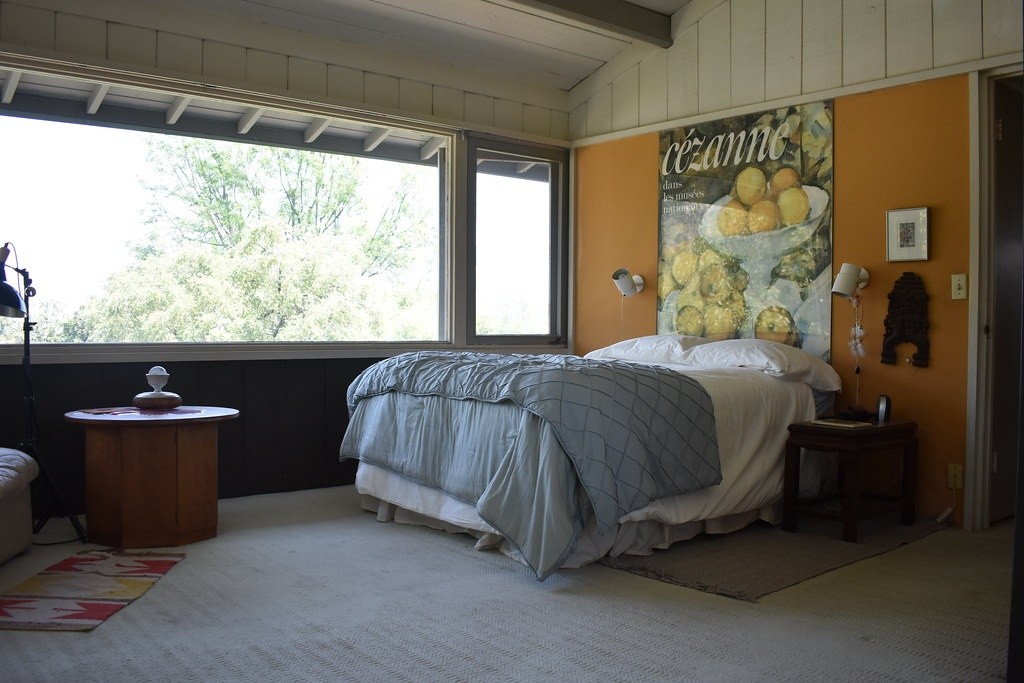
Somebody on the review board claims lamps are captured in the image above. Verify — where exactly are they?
[831,263,870,297]
[0,241,88,545]
[611,268,645,299]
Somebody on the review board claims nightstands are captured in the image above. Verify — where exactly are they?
[782,417,917,542]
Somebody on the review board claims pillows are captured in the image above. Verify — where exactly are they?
[678,338,842,392]
[582,332,695,364]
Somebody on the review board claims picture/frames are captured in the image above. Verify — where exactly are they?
[886,207,930,262]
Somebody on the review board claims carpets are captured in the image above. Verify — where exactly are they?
[0,549,186,634]
[589,512,952,606]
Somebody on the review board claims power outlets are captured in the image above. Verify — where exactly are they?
[950,274,968,300]
[948,461,966,491]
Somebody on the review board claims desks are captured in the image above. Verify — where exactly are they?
[65,406,240,550]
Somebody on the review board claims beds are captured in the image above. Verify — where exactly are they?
[338,331,842,581]
